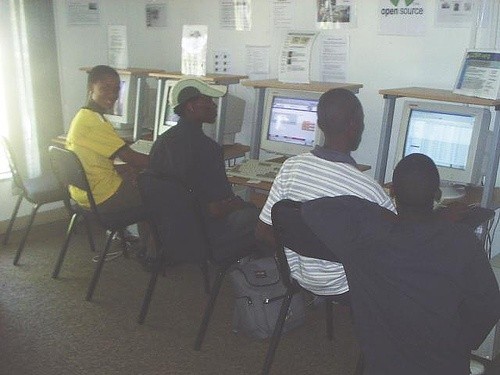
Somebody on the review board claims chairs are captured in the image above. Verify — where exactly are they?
[1,135,63,263]
[133,168,226,348]
[48,146,158,299]
[260,200,352,375]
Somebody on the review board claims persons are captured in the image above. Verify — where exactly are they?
[64,65,158,265]
[253,88,500,375]
[148,78,277,261]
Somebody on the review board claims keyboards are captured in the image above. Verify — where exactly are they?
[130,139,155,156]
[229,159,283,184]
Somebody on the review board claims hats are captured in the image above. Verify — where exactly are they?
[168,76,228,114]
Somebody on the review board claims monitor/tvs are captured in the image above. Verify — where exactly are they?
[260,86,327,157]
[100,73,148,130]
[384,96,492,200]
[158,79,246,147]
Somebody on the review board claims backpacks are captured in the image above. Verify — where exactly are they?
[229,252,306,342]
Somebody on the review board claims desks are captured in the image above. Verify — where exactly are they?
[48,129,499,227]
